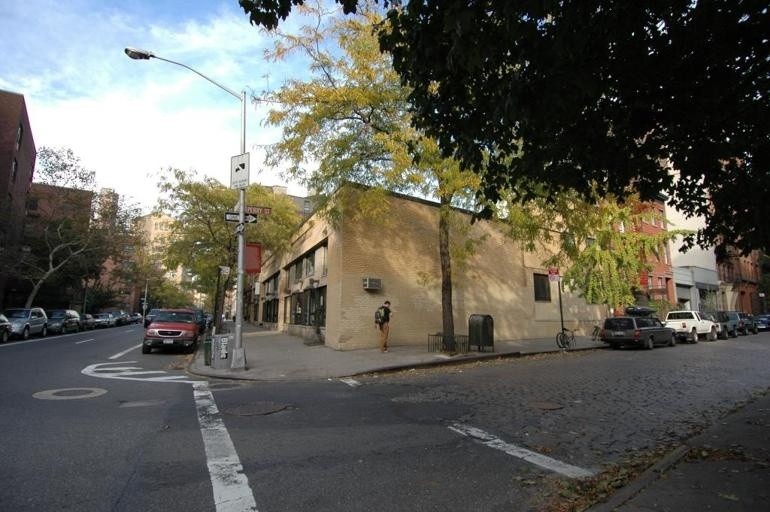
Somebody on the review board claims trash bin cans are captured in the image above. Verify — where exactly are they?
[468,313,495,352]
[211,334,233,369]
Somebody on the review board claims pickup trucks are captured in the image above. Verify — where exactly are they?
[665,310,722,345]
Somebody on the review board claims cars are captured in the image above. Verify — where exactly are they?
[0,312,13,344]
[707,310,770,340]
[81,310,143,331]
[142,308,214,355]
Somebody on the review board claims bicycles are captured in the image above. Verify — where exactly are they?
[592,325,602,341]
[556,328,580,349]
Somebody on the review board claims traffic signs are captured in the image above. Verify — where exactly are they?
[123,45,251,370]
[225,197,271,235]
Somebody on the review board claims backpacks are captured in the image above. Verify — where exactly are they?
[374,306,386,324]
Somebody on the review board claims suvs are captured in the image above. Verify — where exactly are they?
[600,316,677,351]
[45,309,81,335]
[5,306,48,340]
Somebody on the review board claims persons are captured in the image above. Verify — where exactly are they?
[374,300,393,352]
